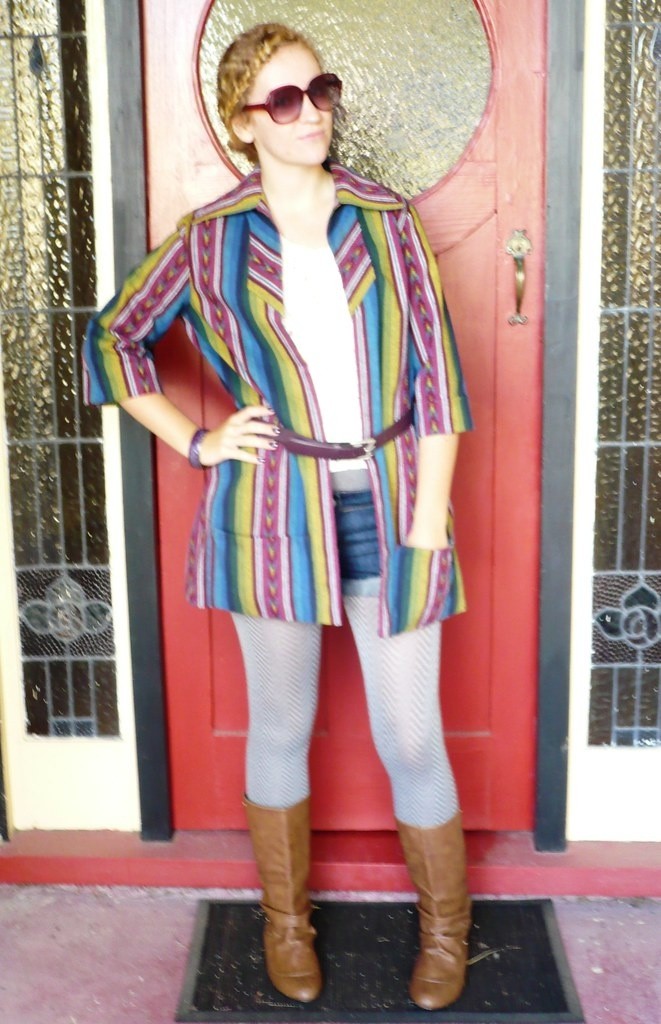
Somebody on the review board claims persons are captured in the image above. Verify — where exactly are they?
[81,24,472,1010]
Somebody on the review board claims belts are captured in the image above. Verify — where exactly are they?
[276,410,414,461]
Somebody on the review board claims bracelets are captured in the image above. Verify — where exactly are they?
[189,429,207,470]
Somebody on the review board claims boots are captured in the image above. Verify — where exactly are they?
[243,793,322,1003]
[394,808,473,1011]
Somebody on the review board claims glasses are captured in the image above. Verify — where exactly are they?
[242,74,343,123]
